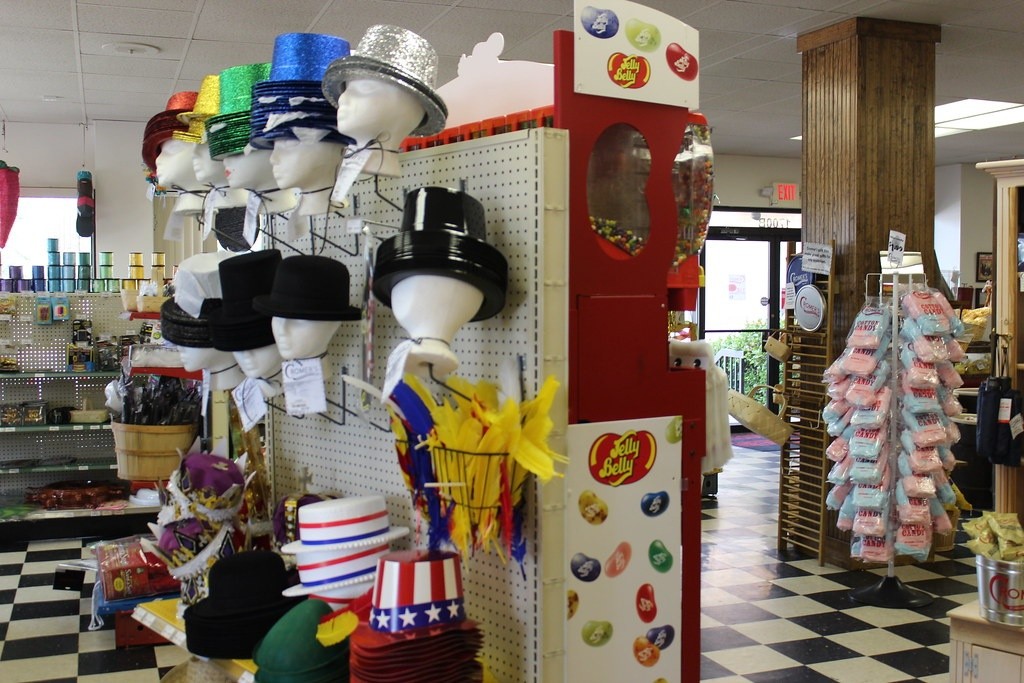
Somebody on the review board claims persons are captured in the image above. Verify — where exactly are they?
[265,99,349,218]
[210,248,289,399]
[322,25,448,183]
[222,114,298,216]
[377,185,507,383]
[162,249,250,393]
[188,121,250,211]
[270,255,360,377]
[155,121,211,215]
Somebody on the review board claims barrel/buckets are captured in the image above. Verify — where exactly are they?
[975,554,1024,629]
[111,420,199,481]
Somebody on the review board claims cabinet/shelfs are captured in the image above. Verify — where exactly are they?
[0,290,232,525]
[950,158,1023,683]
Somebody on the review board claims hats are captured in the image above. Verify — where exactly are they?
[344,547,485,683]
[320,24,448,136]
[253,597,349,683]
[269,490,339,556]
[182,552,307,659]
[171,75,220,144]
[159,252,226,348]
[144,519,234,580]
[250,32,358,149]
[206,249,283,351]
[141,91,199,173]
[204,62,272,162]
[281,493,411,614]
[252,254,362,321]
[372,186,509,322]
[166,435,255,519]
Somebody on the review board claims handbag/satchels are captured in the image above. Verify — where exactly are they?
[764,329,793,363]
[726,384,794,445]
